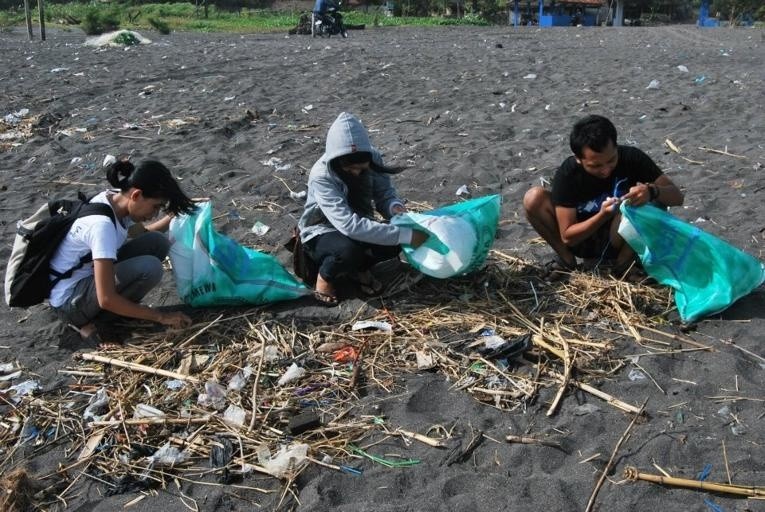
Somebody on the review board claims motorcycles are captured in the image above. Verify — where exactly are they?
[313,1,349,38]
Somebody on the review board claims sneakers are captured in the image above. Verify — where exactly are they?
[113,318,154,327]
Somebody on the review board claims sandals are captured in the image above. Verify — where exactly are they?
[615,261,654,285]
[544,257,577,285]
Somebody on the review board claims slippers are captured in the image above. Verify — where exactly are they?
[313,278,339,307]
[346,272,385,297]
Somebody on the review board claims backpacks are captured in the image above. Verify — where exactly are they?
[4,188,117,308]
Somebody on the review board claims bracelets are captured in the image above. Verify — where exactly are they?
[648,182,659,202]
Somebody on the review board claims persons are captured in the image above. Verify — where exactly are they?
[523,113,684,281]
[282,112,429,309]
[46,160,201,353]
[311,0,339,39]
